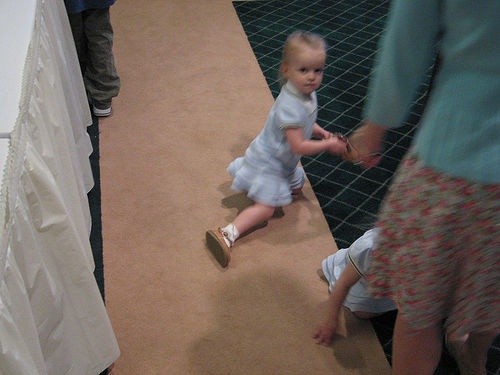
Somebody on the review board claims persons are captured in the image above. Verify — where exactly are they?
[65,0,121,117]
[348,0,500,375]
[309,227,398,347]
[205,31,351,267]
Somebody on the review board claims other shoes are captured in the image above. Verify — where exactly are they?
[92,104,112,117]
[206,230,231,269]
